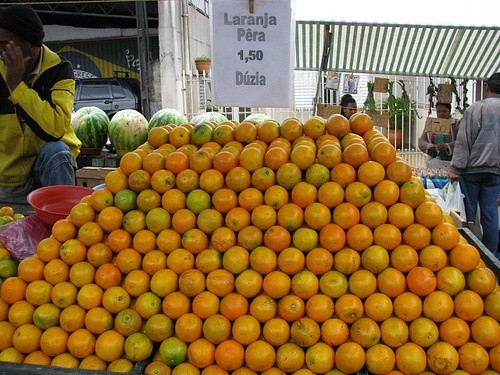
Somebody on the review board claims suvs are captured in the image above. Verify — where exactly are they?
[73,77,141,118]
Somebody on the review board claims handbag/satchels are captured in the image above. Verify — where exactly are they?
[436,181,466,221]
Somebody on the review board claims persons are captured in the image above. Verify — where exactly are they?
[447,72,500,256]
[418,102,461,162]
[0,3,83,198]
[340,94,357,120]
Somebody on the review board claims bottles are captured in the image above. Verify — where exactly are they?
[411,164,449,188]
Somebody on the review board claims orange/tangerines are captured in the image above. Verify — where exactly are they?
[0,112,500,375]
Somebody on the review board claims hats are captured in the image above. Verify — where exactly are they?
[0,4,45,48]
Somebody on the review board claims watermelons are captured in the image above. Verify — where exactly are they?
[189,112,229,124]
[244,113,271,123]
[71,106,110,148]
[108,109,148,156]
[148,108,188,130]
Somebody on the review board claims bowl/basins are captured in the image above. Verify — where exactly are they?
[27,186,94,224]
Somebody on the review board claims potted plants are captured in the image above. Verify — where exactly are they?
[381,95,410,150]
[195,56,211,74]
